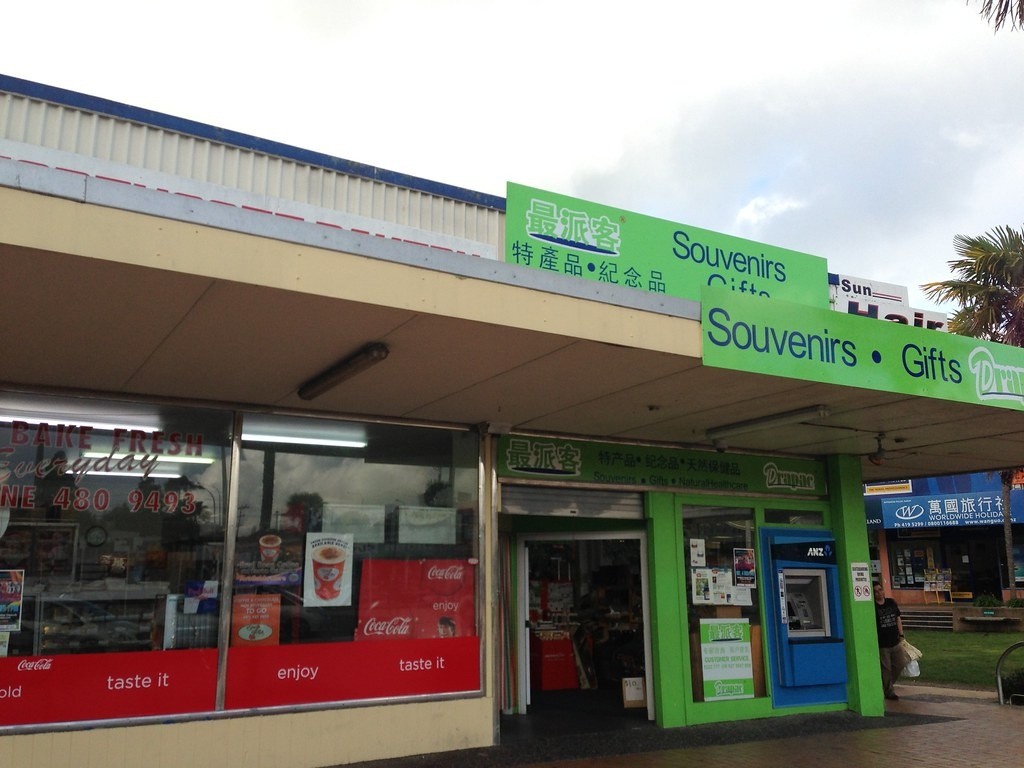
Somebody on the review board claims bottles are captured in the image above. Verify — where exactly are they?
[542,611,570,626]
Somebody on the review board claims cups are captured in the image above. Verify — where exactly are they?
[260,535,281,562]
[309,545,347,601]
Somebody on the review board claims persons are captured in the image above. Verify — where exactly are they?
[438,616,456,638]
[872,580,907,701]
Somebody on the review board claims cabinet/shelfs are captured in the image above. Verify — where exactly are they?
[0,521,170,656]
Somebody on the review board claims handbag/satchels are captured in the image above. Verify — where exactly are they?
[900,639,922,663]
[900,660,920,677]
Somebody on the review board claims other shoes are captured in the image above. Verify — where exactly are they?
[885,694,898,700]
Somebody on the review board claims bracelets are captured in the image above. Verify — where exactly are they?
[899,634,905,638]
